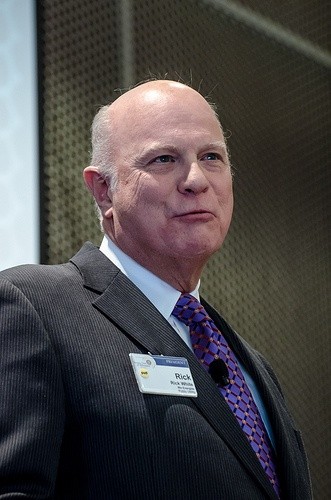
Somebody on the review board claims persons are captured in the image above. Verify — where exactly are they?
[0,80,313,500]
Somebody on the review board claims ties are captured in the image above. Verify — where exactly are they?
[172,293,285,500]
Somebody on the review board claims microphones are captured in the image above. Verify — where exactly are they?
[208,358,230,386]
